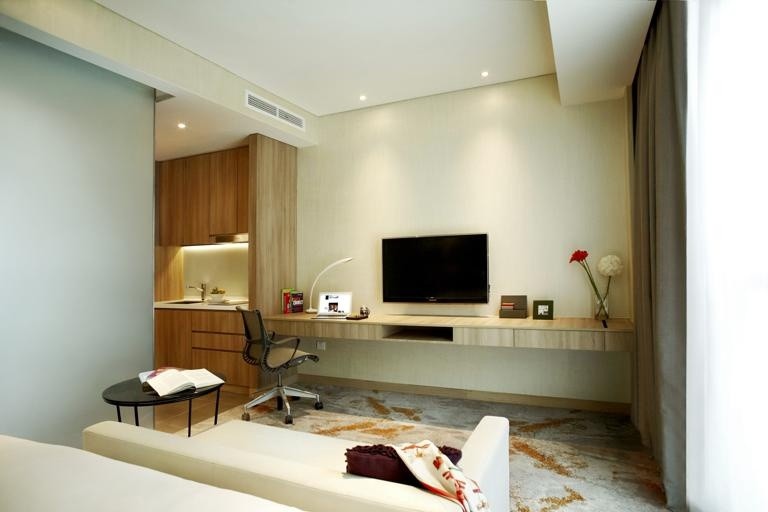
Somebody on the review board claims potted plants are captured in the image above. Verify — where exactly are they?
[208,287,226,303]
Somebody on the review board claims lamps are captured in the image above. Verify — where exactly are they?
[304,258,352,314]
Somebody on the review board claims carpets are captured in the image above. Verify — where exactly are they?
[174,384,686,512]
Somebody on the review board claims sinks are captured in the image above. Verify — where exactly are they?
[167,301,201,304]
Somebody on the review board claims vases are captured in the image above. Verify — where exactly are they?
[593,295,609,321]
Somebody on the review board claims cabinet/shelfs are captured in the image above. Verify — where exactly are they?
[153,308,191,370]
[155,145,209,246]
[263,310,633,355]
[190,298,252,396]
[210,147,249,238]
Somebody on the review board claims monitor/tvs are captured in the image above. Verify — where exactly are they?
[381,232,490,304]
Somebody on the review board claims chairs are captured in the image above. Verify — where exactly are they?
[234,305,325,424]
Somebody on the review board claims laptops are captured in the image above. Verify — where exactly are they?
[311,291,353,320]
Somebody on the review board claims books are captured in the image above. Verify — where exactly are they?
[147,368,225,397]
[146,367,185,380]
[139,371,155,387]
[282,288,304,314]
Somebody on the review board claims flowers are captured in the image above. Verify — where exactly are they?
[569,249,624,318]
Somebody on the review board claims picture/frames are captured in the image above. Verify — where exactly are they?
[533,300,553,320]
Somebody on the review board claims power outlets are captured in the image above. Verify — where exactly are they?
[316,341,326,351]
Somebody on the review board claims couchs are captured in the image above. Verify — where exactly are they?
[82,415,510,512]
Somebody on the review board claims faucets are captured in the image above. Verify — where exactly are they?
[189,283,205,302]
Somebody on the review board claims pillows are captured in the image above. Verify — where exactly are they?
[344,443,462,485]
